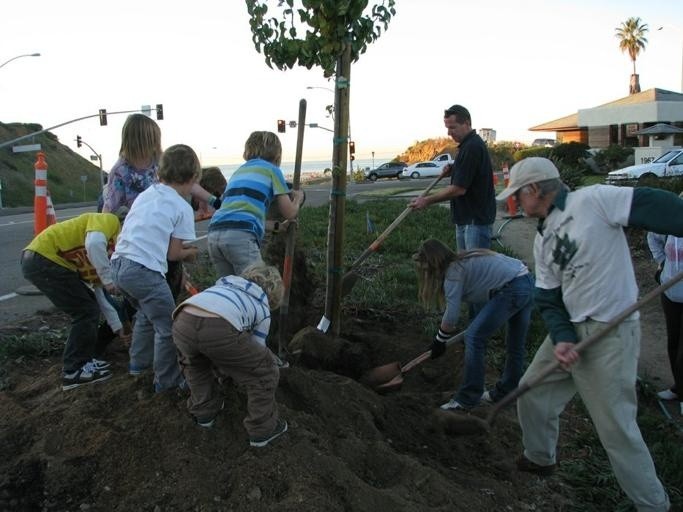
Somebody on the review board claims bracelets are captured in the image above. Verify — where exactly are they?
[271,222,279,235]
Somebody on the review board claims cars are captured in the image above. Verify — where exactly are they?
[362,153,455,181]
[602,149,682,185]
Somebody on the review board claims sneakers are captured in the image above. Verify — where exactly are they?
[62,359,288,447]
[440,392,497,411]
[658,388,683,415]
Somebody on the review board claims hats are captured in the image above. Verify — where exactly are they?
[496,157,560,201]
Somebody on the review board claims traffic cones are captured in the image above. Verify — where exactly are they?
[44,188,55,228]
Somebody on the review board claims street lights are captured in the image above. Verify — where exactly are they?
[0,51,40,74]
[306,85,354,184]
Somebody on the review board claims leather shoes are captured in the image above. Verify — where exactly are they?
[490,454,557,479]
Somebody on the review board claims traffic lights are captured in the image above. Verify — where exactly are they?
[350,156,354,160]
[349,142,353,153]
[277,120,282,133]
[77,133,82,148]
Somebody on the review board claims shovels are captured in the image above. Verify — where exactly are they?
[637,376,682,432]
[274,98,307,386]
[369,331,465,390]
[435,272,683,435]
[340,164,451,297]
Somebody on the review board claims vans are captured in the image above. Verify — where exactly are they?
[188,165,226,212]
[531,139,560,147]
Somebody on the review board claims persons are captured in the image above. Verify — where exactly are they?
[413,237,533,410]
[207,130,305,282]
[404,103,497,321]
[494,156,682,512]
[644,190,683,420]
[19,114,226,392]
[171,262,288,448]
